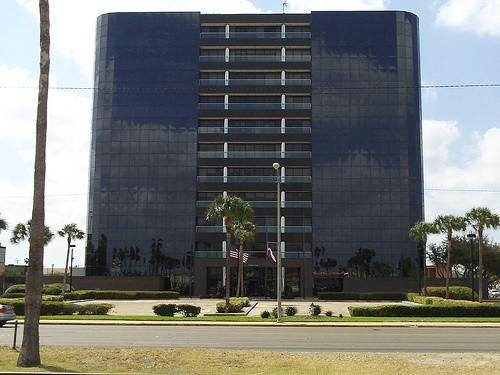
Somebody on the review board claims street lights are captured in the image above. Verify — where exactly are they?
[467,234,476,302]
[70,245,76,292]
[273,163,281,323]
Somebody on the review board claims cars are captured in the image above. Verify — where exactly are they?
[0,304,16,327]
